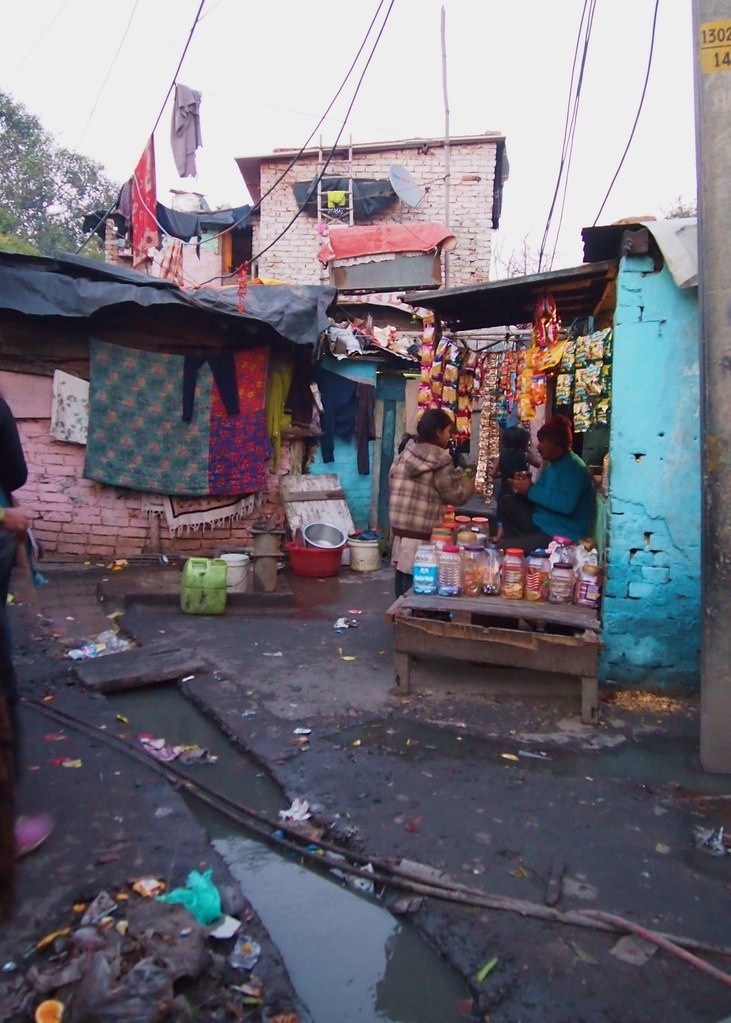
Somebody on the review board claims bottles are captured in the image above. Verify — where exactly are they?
[551,562,577,604]
[501,549,526,598]
[463,545,503,597]
[437,545,463,596]
[525,552,552,603]
[431,505,489,546]
[576,565,599,610]
[412,544,438,594]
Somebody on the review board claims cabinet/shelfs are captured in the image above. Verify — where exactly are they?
[385,587,603,726]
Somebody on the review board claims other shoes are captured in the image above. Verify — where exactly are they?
[11,809,54,857]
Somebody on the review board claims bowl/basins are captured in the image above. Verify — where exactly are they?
[282,540,347,577]
[302,522,346,549]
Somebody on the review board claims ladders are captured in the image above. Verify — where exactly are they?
[317,133,355,285]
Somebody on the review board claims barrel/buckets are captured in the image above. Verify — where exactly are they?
[346,533,381,572]
[220,553,249,594]
[180,557,228,614]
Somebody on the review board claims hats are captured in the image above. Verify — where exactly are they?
[536,413,573,449]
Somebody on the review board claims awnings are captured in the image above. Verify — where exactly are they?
[397,258,617,335]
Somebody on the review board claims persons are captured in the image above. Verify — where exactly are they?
[496,414,595,553]
[389,407,478,602]
[0,390,55,922]
[396,433,418,456]
[491,426,543,544]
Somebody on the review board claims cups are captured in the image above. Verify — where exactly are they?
[515,471,532,480]
